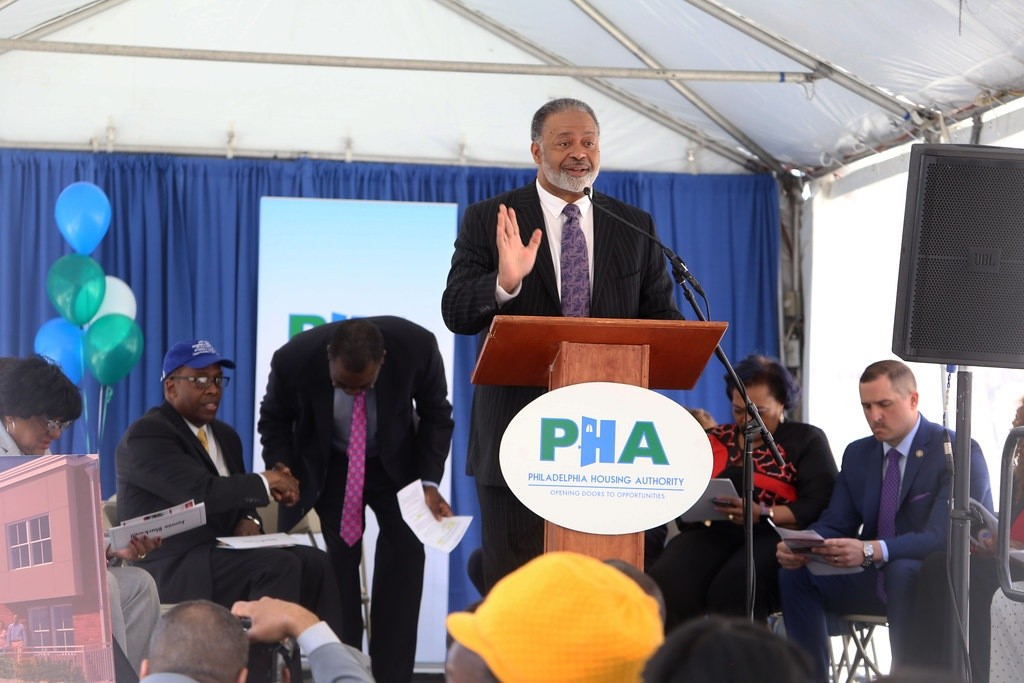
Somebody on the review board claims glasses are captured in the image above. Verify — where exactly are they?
[169,376,230,389]
[42,415,73,432]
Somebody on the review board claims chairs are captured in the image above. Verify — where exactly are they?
[100,492,369,683]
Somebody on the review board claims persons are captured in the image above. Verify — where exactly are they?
[0,355,162,683]
[7,615,27,663]
[258,316,452,683]
[778,360,1024,683]
[645,356,839,621]
[140,595,376,683]
[444,551,817,683]
[114,339,343,640]
[441,99,686,589]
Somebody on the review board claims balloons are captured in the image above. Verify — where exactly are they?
[84,314,144,385]
[77,275,137,351]
[46,253,106,326]
[34,318,87,384]
[54,181,112,256]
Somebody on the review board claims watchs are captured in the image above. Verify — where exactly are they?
[863,541,873,568]
[759,502,772,523]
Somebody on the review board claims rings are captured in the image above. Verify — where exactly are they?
[835,557,838,563]
[137,554,146,559]
[729,514,734,520]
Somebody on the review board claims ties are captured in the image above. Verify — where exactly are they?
[876,449,904,603]
[560,204,591,318]
[339,393,367,549]
[198,429,209,452]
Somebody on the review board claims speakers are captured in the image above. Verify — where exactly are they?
[892,143,1024,369]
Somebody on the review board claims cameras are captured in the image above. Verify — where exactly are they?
[238,617,277,683]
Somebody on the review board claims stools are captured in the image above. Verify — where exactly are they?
[829,613,887,683]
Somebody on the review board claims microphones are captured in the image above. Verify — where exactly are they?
[584,187,706,297]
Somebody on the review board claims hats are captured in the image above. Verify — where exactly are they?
[445,550,664,683]
[161,340,236,380]
